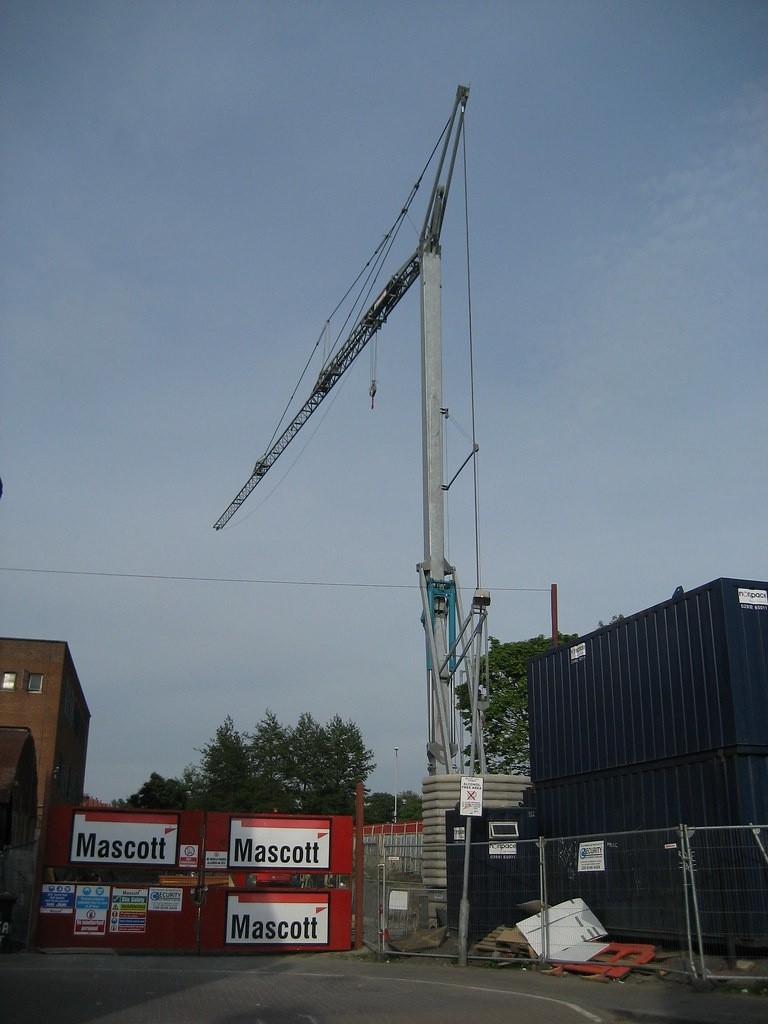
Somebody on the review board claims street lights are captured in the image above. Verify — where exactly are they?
[394,747,398,824]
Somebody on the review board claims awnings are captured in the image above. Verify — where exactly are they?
[0,730,30,803]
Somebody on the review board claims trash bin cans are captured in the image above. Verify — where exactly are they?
[0,891,18,949]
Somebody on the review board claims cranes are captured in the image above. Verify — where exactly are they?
[212,83,531,930]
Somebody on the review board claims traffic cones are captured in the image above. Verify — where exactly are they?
[376,905,394,942]
